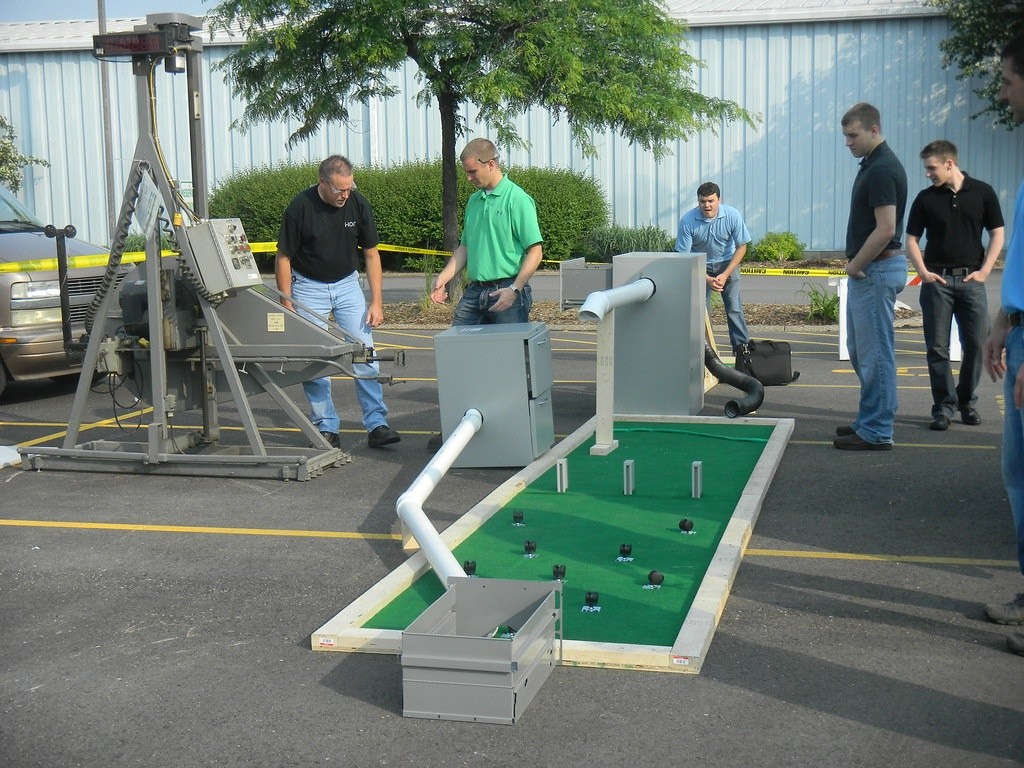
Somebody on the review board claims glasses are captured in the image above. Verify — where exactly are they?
[324,178,358,196]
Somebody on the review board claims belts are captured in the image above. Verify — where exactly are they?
[473,275,518,287]
[926,265,981,277]
[872,248,903,262]
[1007,311,1024,327]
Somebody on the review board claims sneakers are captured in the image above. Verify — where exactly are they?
[833,432,893,451]
[309,431,341,450]
[368,424,401,447]
[836,425,857,436]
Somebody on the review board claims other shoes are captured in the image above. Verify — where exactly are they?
[1006,632,1024,655]
[428,431,442,450]
[983,592,1024,626]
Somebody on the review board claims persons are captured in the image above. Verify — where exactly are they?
[904,140,1005,430]
[674,182,752,356]
[833,102,908,450]
[982,37,1024,655]
[426,138,544,450]
[275,156,401,449]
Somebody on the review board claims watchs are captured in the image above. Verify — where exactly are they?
[509,284,520,296]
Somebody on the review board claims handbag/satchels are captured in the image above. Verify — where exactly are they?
[732,339,800,386]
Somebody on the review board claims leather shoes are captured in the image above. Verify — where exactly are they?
[957,407,982,425]
[929,415,951,431]
